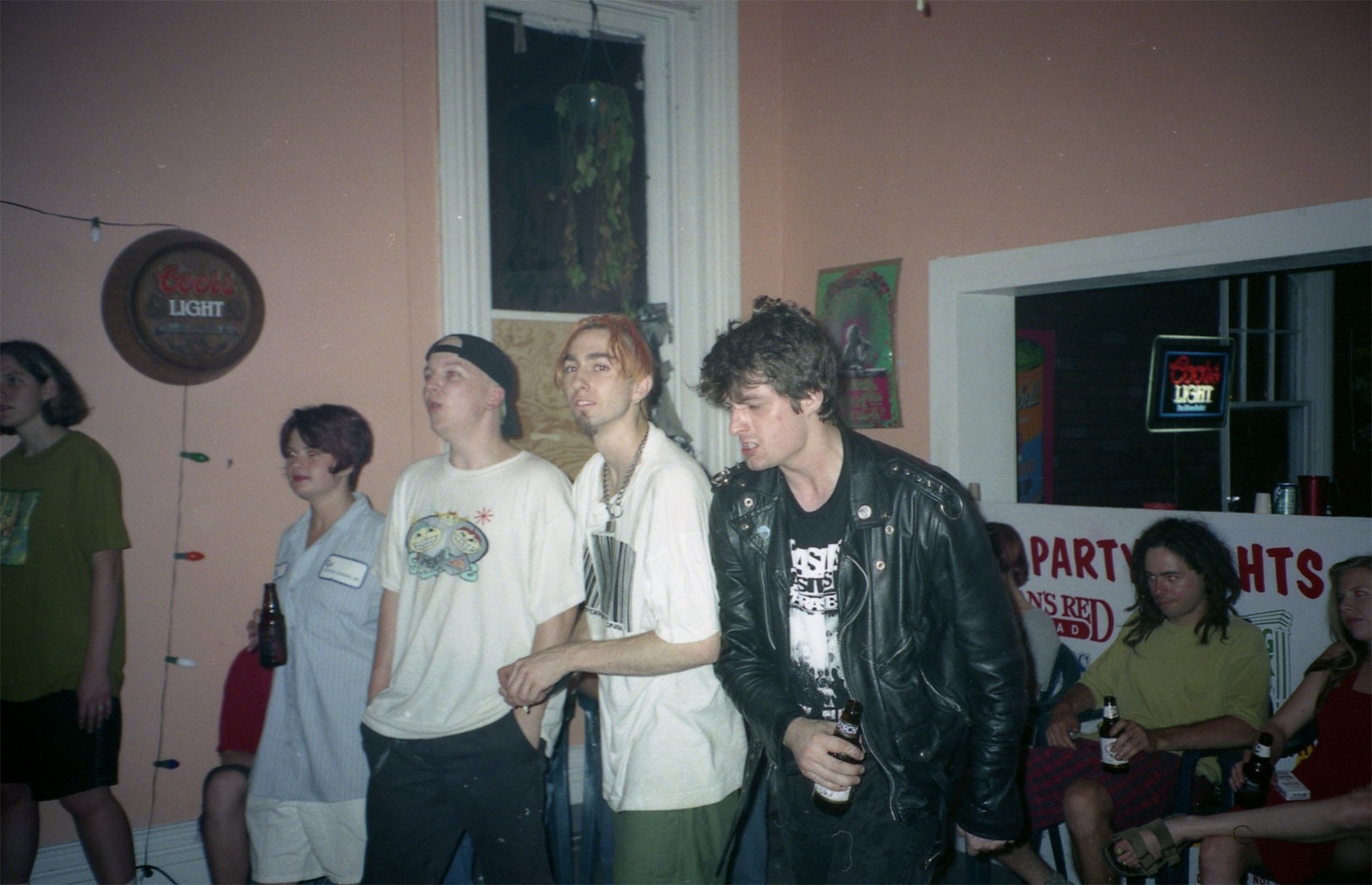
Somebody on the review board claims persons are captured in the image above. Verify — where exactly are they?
[360,334,585,885]
[245,403,384,885]
[202,647,274,885]
[0,339,138,885]
[995,517,1272,885]
[700,305,1024,885]
[988,519,1060,696]
[497,315,748,885]
[1104,556,1372,885]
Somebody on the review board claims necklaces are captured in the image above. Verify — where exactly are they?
[602,424,648,533]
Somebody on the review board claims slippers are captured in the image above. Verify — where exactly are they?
[1102,815,1193,879]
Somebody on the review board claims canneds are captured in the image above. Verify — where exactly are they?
[839,368,893,425]
[1272,483,1297,515]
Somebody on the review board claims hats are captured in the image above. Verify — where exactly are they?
[424,333,524,439]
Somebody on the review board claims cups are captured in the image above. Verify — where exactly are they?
[1298,475,1330,516]
[1254,493,1271,513]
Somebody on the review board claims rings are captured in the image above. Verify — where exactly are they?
[96,704,106,710]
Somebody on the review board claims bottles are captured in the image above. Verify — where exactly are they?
[1100,697,1131,773]
[258,583,286,668]
[1221,732,1273,812]
[812,700,862,814]
